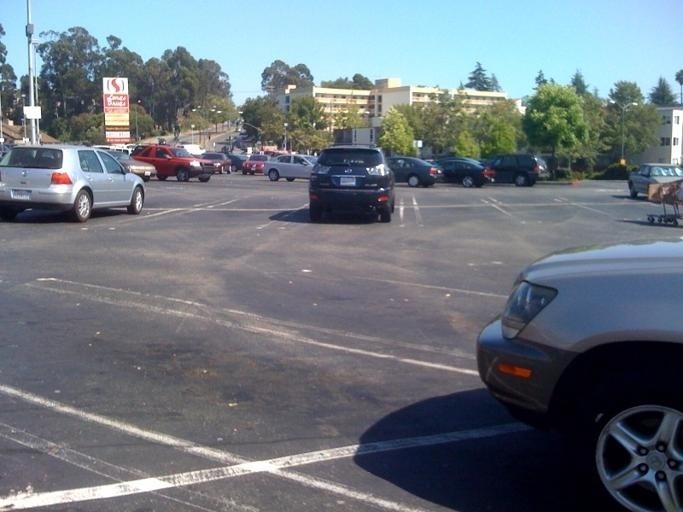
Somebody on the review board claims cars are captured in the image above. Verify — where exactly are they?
[627,162,682,200]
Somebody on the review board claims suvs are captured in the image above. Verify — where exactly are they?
[477,237,682,511]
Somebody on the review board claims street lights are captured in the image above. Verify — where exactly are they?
[622,100,637,165]
[2,95,295,150]
